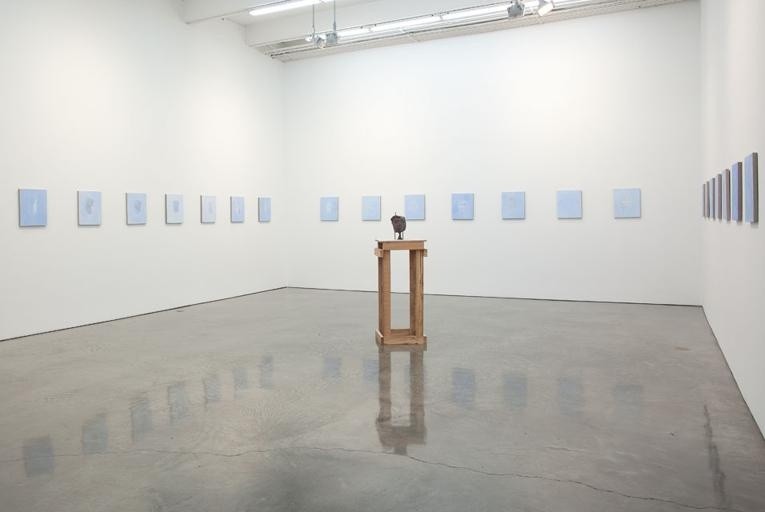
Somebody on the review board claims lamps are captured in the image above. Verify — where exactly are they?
[537,3,554,17]
[246,2,320,16]
[254,2,526,52]
